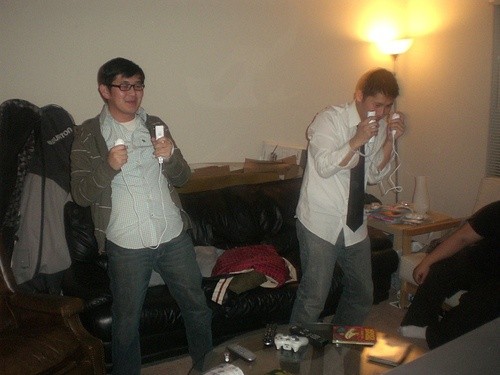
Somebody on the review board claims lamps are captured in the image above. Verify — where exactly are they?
[376,38,413,206]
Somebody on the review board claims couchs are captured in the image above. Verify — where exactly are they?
[398,164,500,313]
[63,175,400,368]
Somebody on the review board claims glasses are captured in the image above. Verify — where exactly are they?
[110,83,145,91]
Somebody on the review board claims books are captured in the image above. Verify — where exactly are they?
[331,326,376,346]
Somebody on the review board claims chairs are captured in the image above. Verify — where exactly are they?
[0,239,105,372]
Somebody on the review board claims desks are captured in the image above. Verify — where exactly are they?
[367,207,464,310]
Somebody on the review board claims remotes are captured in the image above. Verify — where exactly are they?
[289,325,329,347]
[227,344,256,361]
[262,323,277,347]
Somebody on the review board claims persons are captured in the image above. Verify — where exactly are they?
[69,56,214,375]
[397,200,499,350]
[289,68,407,327]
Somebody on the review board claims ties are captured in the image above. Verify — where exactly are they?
[346,126,365,233]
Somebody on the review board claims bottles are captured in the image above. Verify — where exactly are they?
[412,175,430,213]
